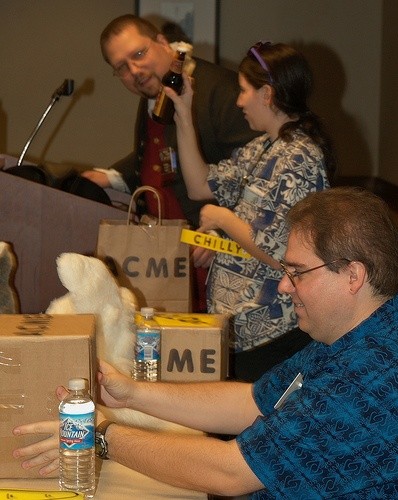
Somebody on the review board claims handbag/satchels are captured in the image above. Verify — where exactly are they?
[97,187,192,315]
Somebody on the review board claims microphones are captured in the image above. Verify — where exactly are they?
[14,77,75,167]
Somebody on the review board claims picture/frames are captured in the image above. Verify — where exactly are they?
[135,0,220,65]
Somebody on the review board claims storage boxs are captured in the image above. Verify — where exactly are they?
[0,314,98,480]
[152,311,229,384]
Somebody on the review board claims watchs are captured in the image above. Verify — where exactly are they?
[95,420,116,460]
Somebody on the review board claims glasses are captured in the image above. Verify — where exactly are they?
[248,40,274,83]
[279,258,352,287]
[112,34,158,77]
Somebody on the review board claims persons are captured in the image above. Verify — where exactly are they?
[13,188,398,500]
[80,13,254,313]
[165,42,331,384]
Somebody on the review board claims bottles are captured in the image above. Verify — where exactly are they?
[131,307,160,382]
[153,46,186,124]
[58,379,98,500]
